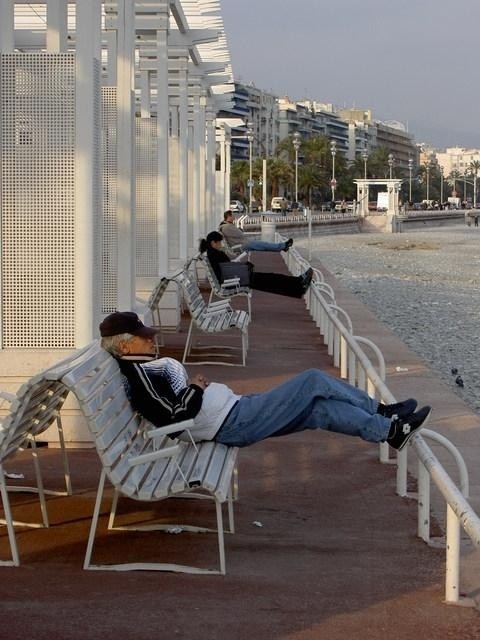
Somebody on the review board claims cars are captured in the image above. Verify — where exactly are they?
[248,202,260,213]
[320,200,356,212]
[230,199,244,213]
[368,202,377,210]
[271,197,305,212]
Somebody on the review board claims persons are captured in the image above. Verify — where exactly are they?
[221,210,293,252]
[257,198,262,212]
[330,200,338,214]
[99,312,433,452]
[291,198,298,215]
[279,198,288,215]
[408,200,476,210]
[200,232,313,299]
[340,199,347,214]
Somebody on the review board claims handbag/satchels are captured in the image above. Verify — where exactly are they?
[220,262,250,286]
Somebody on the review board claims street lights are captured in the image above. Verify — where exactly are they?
[292,131,302,204]
[425,162,431,199]
[408,159,414,203]
[361,149,368,179]
[245,122,254,214]
[440,166,445,204]
[388,154,394,179]
[330,140,337,209]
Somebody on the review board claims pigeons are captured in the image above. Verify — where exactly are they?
[451,367,458,376]
[456,374,464,388]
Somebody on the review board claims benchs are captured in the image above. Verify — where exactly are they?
[135,265,173,358]
[186,232,251,308]
[1,340,96,570]
[169,266,249,368]
[45,341,240,575]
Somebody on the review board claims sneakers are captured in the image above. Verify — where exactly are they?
[384,399,417,417]
[284,239,293,252]
[387,406,431,451]
[300,268,312,293]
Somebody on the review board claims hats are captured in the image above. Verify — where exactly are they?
[99,312,158,338]
